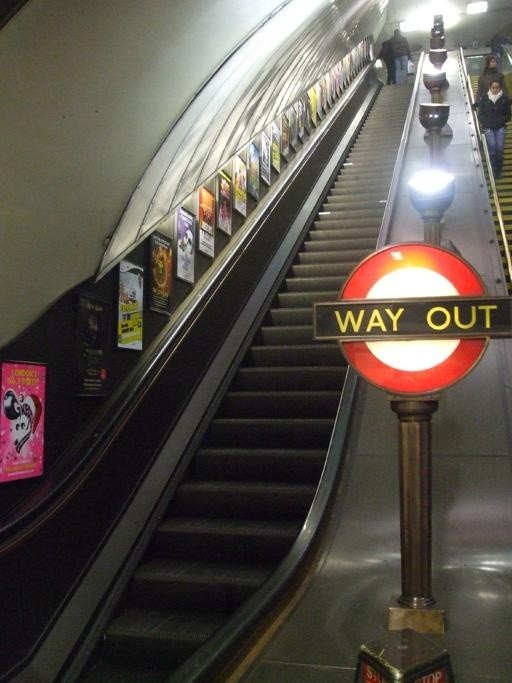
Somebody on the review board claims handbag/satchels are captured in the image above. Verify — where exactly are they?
[407,59,416,75]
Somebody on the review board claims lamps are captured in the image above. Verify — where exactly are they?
[420,102,449,169]
[423,71,446,103]
[429,14,445,50]
[407,171,455,246]
[429,49,447,71]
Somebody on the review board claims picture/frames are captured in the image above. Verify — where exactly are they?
[0,359,49,485]
[233,37,372,218]
[217,172,233,237]
[176,206,196,285]
[147,230,173,317]
[198,185,216,259]
[114,258,145,351]
[70,294,114,400]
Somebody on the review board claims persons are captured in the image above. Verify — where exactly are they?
[390,29,413,85]
[474,53,508,106]
[377,41,396,85]
[476,79,512,180]
